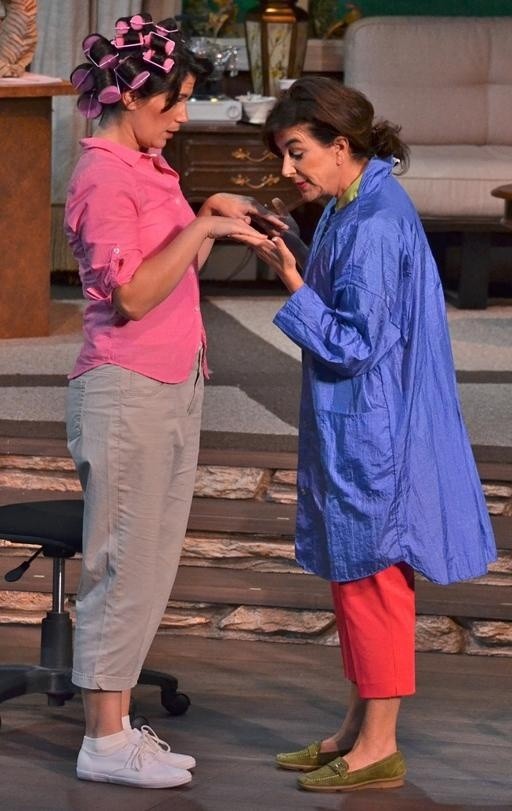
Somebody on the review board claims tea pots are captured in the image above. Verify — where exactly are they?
[188,37,241,84]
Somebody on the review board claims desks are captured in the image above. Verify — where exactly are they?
[0,71,80,339]
[177,120,332,246]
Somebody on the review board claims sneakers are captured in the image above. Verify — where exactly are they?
[276,740,350,772]
[297,751,407,793]
[77,728,197,790]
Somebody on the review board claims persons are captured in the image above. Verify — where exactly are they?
[63,12,290,790]
[228,77,499,794]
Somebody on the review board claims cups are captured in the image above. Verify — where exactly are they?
[279,79,296,99]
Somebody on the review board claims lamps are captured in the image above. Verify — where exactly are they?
[243,0,309,97]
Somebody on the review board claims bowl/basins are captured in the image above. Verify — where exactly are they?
[236,96,278,122]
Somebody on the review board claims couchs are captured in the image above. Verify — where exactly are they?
[343,16,512,310]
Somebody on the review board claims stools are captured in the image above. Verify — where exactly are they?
[0,500,191,730]
